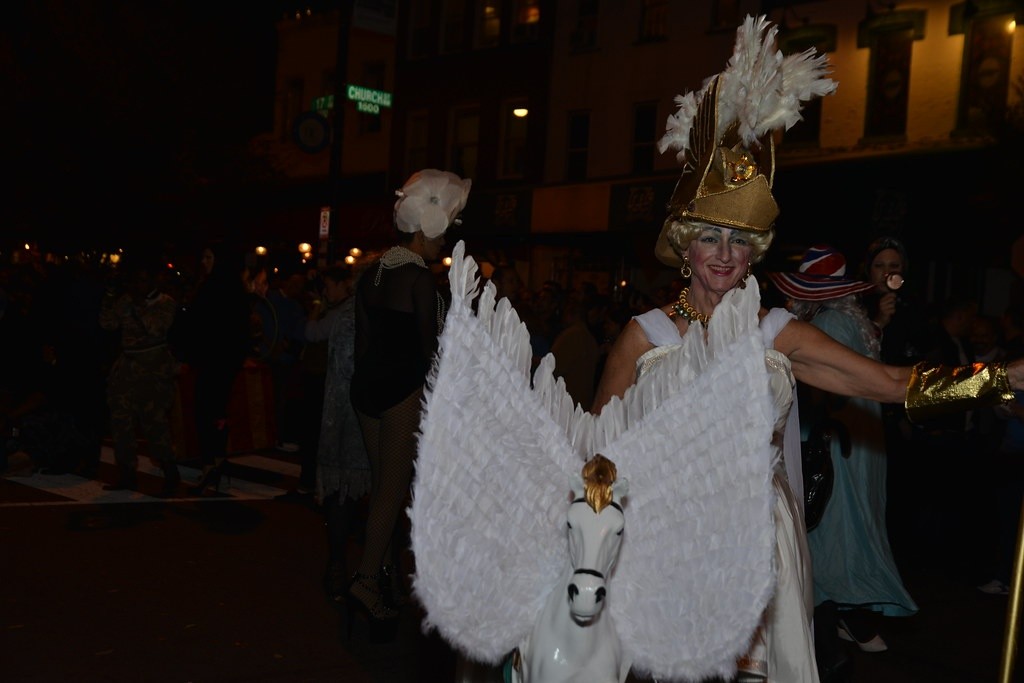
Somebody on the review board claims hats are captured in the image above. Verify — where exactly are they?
[768,245,879,302]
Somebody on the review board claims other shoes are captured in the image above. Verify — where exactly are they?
[102,469,137,490]
[286,488,315,504]
[164,473,179,499]
[837,615,887,650]
[977,580,1011,595]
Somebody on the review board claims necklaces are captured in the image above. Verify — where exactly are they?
[670,288,711,329]
[377,244,445,333]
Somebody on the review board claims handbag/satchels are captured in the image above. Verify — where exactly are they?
[800,436,834,532]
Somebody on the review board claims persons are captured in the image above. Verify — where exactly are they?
[591,223,1024,683]
[768,244,919,652]
[857,233,1024,595]
[97,168,683,643]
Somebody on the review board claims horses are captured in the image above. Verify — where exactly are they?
[510,452,631,683]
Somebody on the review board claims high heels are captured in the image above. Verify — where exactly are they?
[216,459,231,487]
[189,467,222,497]
